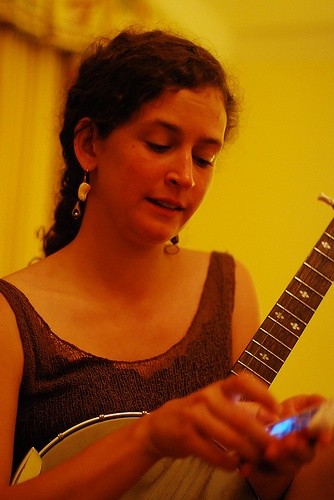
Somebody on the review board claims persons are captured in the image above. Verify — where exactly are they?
[0,21,334,500]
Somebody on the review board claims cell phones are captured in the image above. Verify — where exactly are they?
[263,402,328,439]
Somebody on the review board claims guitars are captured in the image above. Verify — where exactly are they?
[12,193,334,500]
